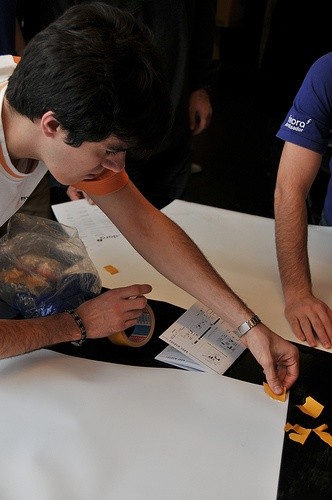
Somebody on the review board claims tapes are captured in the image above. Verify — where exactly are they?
[110,302,156,349]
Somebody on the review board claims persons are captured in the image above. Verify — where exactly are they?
[134,1,216,145]
[0,4,301,394]
[275,51,332,350]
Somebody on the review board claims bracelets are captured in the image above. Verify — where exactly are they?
[64,307,85,346]
[233,314,260,338]
